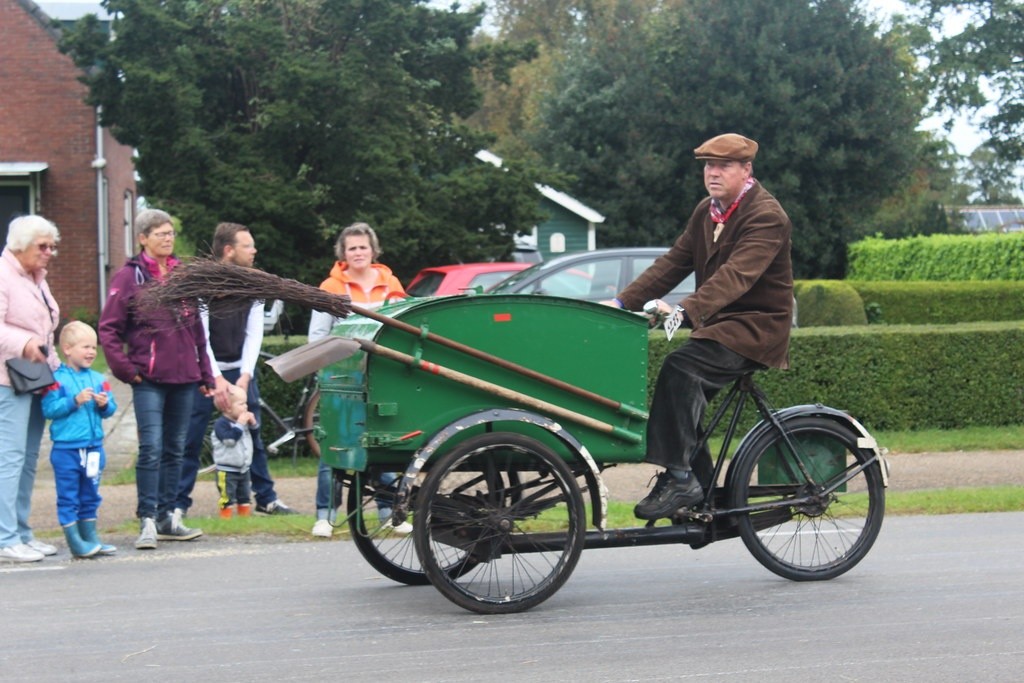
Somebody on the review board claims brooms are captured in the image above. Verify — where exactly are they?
[122,233,620,410]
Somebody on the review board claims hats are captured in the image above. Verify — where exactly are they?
[693,132,759,160]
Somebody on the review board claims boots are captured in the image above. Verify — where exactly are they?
[77,519,117,553]
[63,523,101,558]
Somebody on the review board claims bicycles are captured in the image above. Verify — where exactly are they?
[197,351,322,475]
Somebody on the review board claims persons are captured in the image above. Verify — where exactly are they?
[41,319,118,558]
[172,222,299,518]
[211,387,260,518]
[600,133,794,518]
[308,222,413,537]
[98,208,218,549]
[0,215,60,562]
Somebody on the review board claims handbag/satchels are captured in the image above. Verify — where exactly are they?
[5,355,55,396]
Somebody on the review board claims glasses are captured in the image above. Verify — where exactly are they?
[40,244,59,253]
[142,231,177,240]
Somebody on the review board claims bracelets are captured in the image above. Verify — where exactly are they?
[677,303,688,323]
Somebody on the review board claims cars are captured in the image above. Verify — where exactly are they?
[410,265,618,297]
[486,248,797,332]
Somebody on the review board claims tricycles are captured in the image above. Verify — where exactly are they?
[321,293,888,615]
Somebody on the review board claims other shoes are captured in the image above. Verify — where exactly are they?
[26,538,57,556]
[382,517,413,534]
[312,519,335,538]
[254,499,297,516]
[0,542,45,562]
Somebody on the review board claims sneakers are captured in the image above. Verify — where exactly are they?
[635,471,704,520]
[135,517,158,549]
[155,514,203,541]
